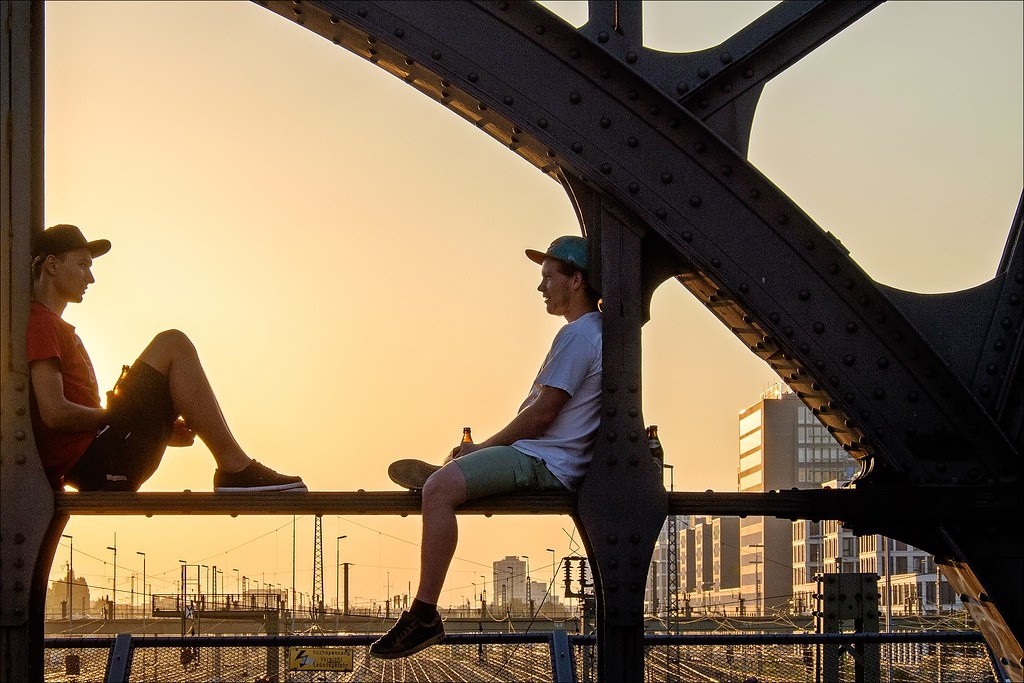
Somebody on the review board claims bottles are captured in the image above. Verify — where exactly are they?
[459,426,473,451]
[646,423,663,486]
[112,365,130,410]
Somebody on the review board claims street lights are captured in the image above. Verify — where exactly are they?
[521,555,530,618]
[233,569,239,603]
[546,548,555,617]
[507,566,513,610]
[179,560,186,635]
[561,586,572,617]
[480,576,486,600]
[201,564,208,610]
[461,595,465,618]
[136,552,145,618]
[62,534,73,637]
[472,583,476,617]
[106,547,116,619]
[218,571,223,610]
[245,577,310,619]
[336,535,347,611]
[493,572,499,617]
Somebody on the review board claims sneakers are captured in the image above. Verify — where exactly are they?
[213,458,308,494]
[388,459,443,490]
[370,605,446,660]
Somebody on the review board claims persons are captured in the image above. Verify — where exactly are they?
[26,223,309,494]
[370,235,603,658]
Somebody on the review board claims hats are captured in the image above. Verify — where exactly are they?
[34,224,112,271]
[524,235,588,275]
